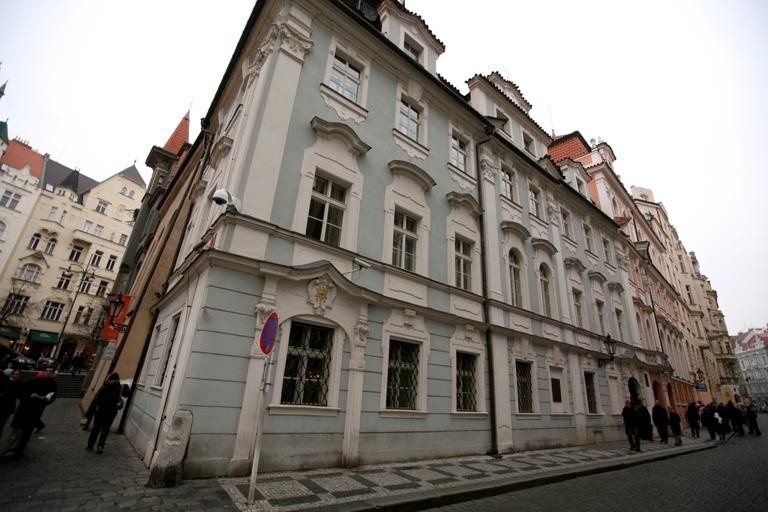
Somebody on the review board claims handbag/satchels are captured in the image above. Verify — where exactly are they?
[115,397,124,410]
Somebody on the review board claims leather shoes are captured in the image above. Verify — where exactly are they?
[630,439,681,451]
[34,424,46,434]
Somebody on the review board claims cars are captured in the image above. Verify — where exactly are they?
[1,346,35,370]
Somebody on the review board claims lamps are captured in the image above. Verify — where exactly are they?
[597,332,618,368]
[107,291,129,334]
[690,367,704,384]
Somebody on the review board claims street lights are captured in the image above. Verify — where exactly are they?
[48,253,96,366]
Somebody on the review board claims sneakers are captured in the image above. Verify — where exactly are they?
[691,431,762,441]
[85,446,103,454]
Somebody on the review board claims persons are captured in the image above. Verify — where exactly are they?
[58,352,84,375]
[0,367,57,457]
[622,396,762,452]
[84,372,129,454]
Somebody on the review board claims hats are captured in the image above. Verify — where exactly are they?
[625,400,630,403]
